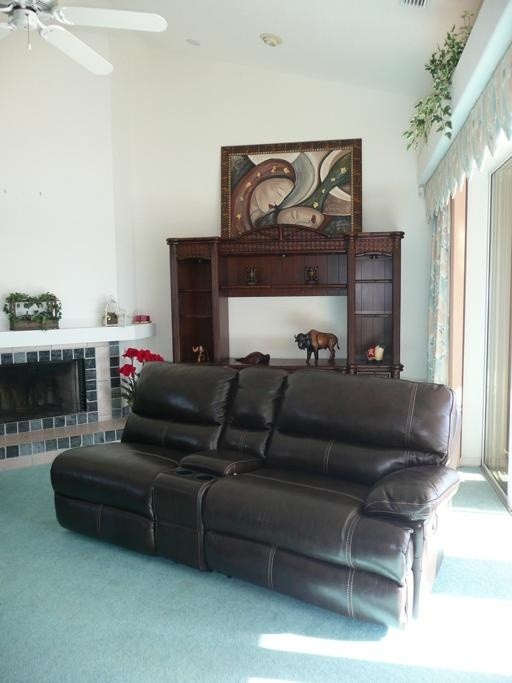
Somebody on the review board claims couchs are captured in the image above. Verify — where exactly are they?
[51,361,461,630]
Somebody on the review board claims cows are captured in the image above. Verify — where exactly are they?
[294,329,340,363]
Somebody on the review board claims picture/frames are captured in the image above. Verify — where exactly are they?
[221,138,363,240]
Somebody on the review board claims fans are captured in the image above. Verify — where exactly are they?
[1,1,169,76]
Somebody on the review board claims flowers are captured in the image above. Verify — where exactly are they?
[118,348,166,410]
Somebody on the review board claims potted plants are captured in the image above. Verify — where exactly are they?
[3,292,63,330]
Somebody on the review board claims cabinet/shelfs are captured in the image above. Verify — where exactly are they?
[167,231,406,380]
